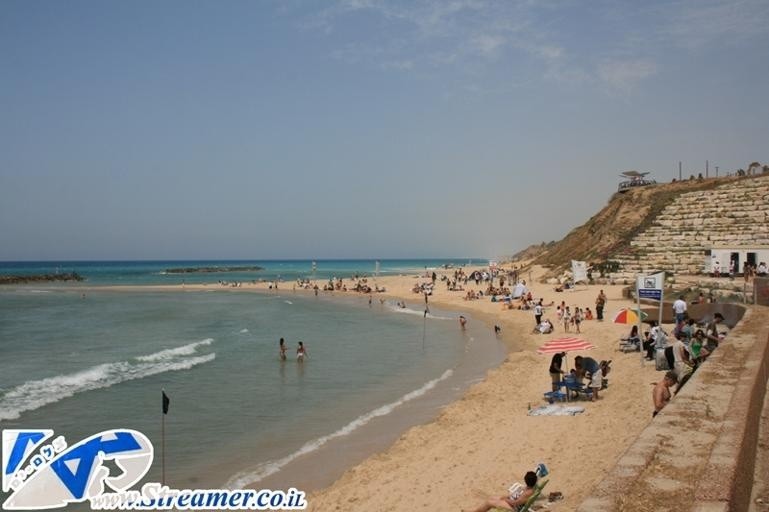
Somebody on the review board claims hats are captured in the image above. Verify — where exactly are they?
[714,312,725,320]
[664,371,680,384]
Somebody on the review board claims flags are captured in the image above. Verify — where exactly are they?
[162,391,172,414]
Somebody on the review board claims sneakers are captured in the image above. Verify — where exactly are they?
[548,492,564,502]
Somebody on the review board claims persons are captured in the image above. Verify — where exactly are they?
[414,258,768,418]
[460,470,538,512]
[280,337,290,359]
[218,270,409,311]
[297,341,309,363]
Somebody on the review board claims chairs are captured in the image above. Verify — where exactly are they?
[490,478,551,511]
[543,374,607,404]
